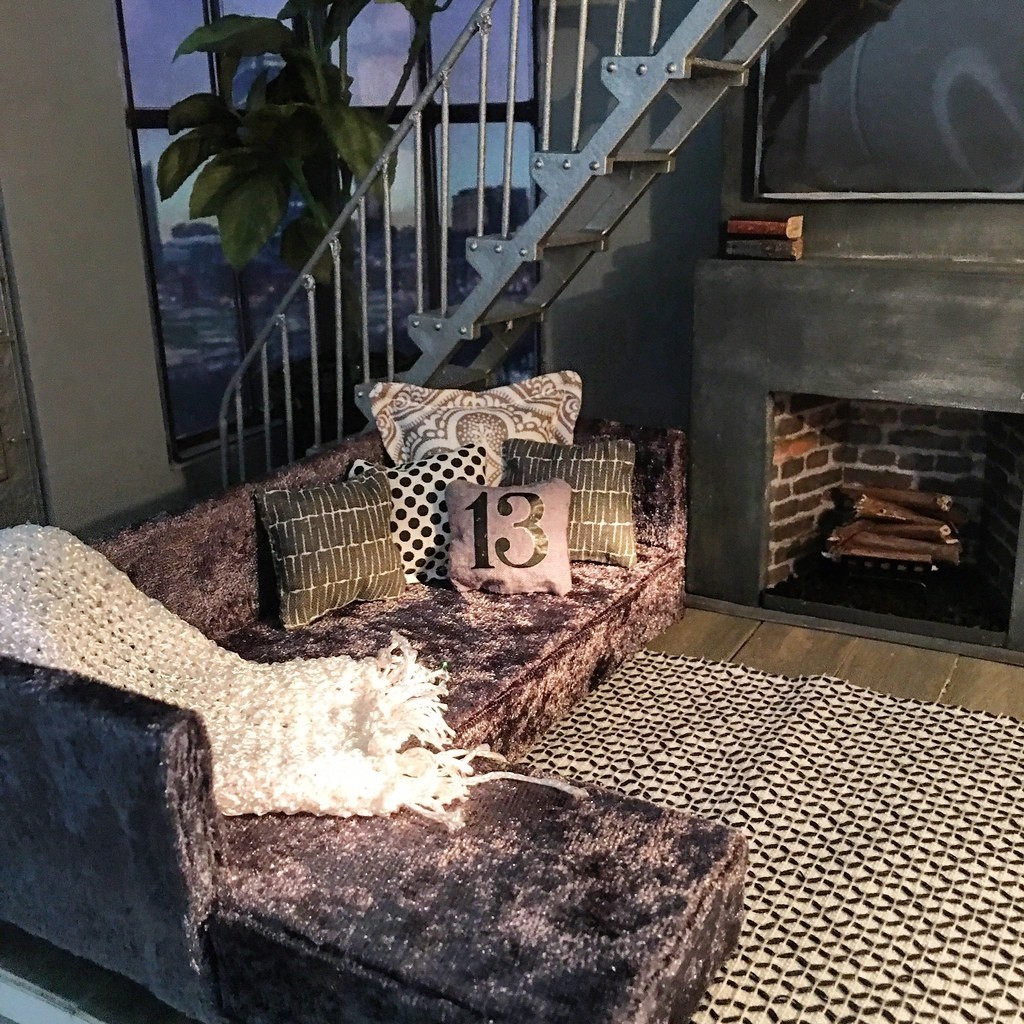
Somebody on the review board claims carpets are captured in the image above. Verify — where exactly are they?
[509,647,1024,1024]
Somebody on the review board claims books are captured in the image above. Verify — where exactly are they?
[726,214,803,260]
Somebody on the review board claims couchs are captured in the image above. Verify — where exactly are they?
[0,416,749,1024]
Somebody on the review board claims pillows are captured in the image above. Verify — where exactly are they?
[445,479,572,597]
[499,438,636,571]
[347,444,487,584]
[367,370,582,487]
[253,472,406,628]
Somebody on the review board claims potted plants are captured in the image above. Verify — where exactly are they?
[155,1,453,466]
